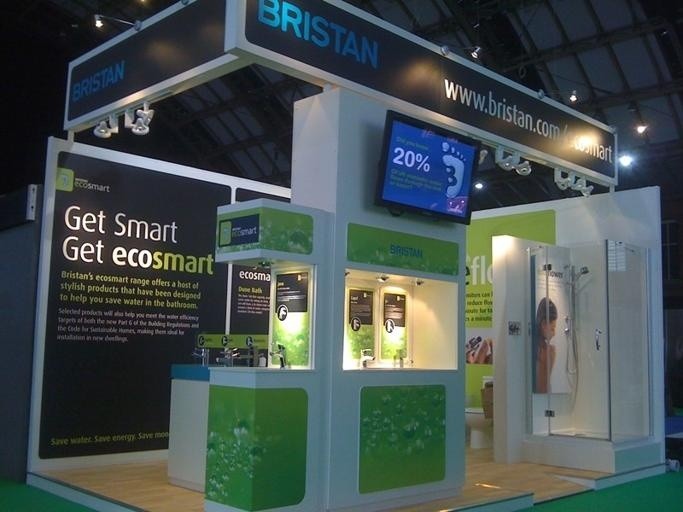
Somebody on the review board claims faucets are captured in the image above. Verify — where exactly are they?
[359,348,375,368]
[191,343,287,368]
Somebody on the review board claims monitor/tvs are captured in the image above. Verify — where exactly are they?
[374,109,482,225]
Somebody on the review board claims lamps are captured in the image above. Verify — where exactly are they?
[493,146,532,177]
[124,110,150,136]
[135,103,154,125]
[93,113,119,138]
[553,167,593,198]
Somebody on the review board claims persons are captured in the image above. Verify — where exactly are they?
[465,337,493,365]
[535,297,559,393]
